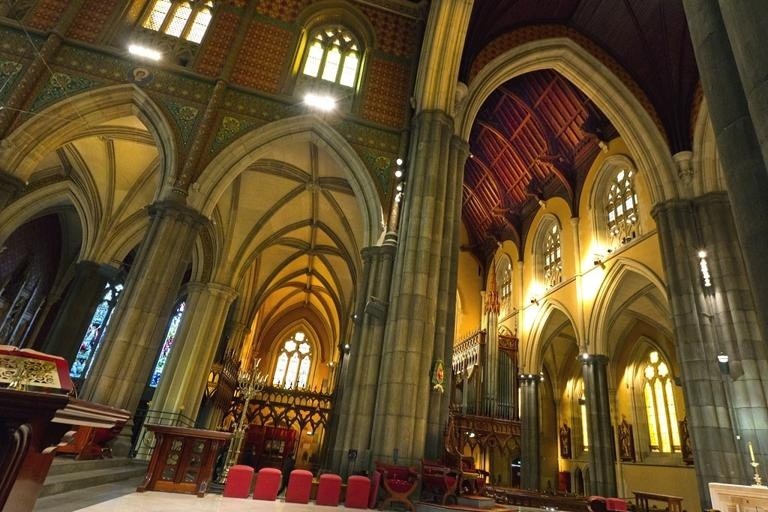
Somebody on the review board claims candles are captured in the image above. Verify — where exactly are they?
[748,441,755,461]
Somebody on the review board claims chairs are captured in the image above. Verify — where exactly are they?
[376,414,491,512]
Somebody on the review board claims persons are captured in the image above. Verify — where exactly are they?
[211,401,251,481]
